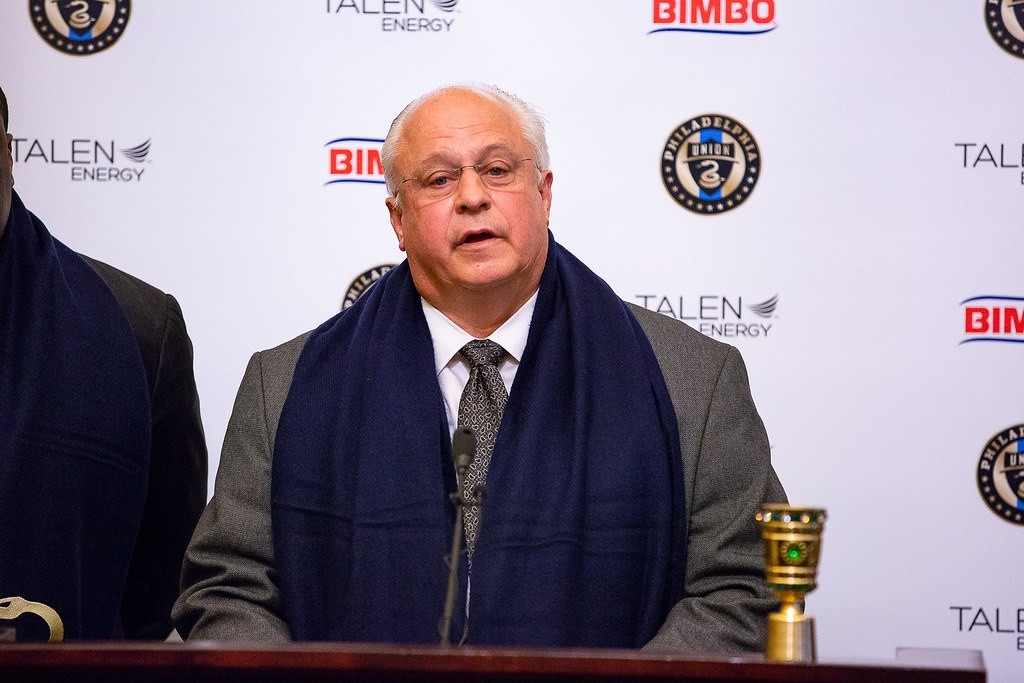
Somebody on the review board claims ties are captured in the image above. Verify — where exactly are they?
[458,336,511,575]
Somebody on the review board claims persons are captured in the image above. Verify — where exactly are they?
[0,82,209,644]
[172,82,805,656]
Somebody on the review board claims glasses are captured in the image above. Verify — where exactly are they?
[395,156,543,191]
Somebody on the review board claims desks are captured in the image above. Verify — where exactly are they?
[0,642,990,683]
